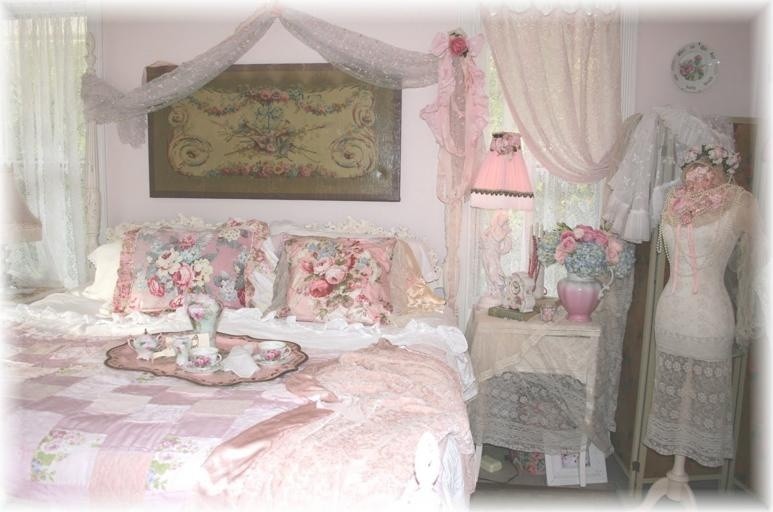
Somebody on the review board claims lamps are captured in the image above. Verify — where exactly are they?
[468,130,536,311]
[5,161,42,243]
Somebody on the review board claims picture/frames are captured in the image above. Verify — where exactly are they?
[544,429,609,487]
[144,59,404,204]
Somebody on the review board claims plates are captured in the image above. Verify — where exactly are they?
[181,366,225,377]
[253,355,292,368]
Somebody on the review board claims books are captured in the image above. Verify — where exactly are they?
[487,304,540,322]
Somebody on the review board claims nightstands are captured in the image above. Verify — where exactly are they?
[466,297,601,488]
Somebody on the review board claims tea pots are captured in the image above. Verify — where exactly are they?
[125,331,164,361]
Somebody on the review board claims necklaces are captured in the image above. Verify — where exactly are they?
[655,181,746,278]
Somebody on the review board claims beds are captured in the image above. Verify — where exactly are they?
[1,217,480,507]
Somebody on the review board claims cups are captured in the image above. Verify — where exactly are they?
[189,346,222,368]
[258,341,292,361]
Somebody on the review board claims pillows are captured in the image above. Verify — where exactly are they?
[112,216,278,318]
[262,235,406,327]
[83,241,123,302]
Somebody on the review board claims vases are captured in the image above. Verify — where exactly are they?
[553,271,612,323]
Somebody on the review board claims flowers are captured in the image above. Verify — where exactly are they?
[541,220,622,278]
